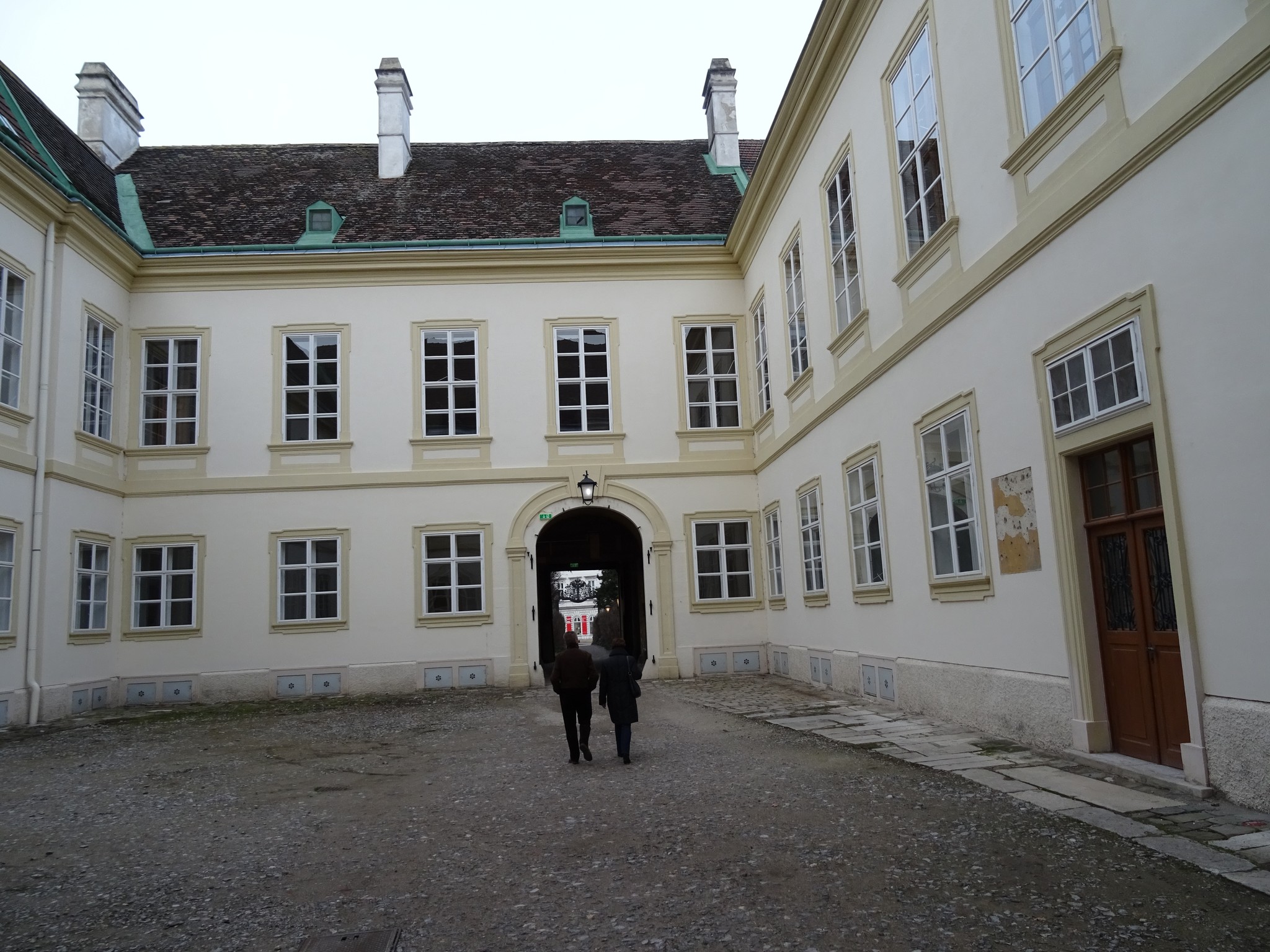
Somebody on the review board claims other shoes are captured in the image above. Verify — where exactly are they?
[569,758,578,764]
[580,743,592,761]
[623,756,630,764]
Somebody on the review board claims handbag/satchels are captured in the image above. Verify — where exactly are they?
[626,654,642,698]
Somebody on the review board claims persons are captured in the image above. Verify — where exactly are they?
[599,638,642,765]
[550,631,599,764]
[574,628,580,635]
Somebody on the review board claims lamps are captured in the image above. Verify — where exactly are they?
[577,469,598,506]
